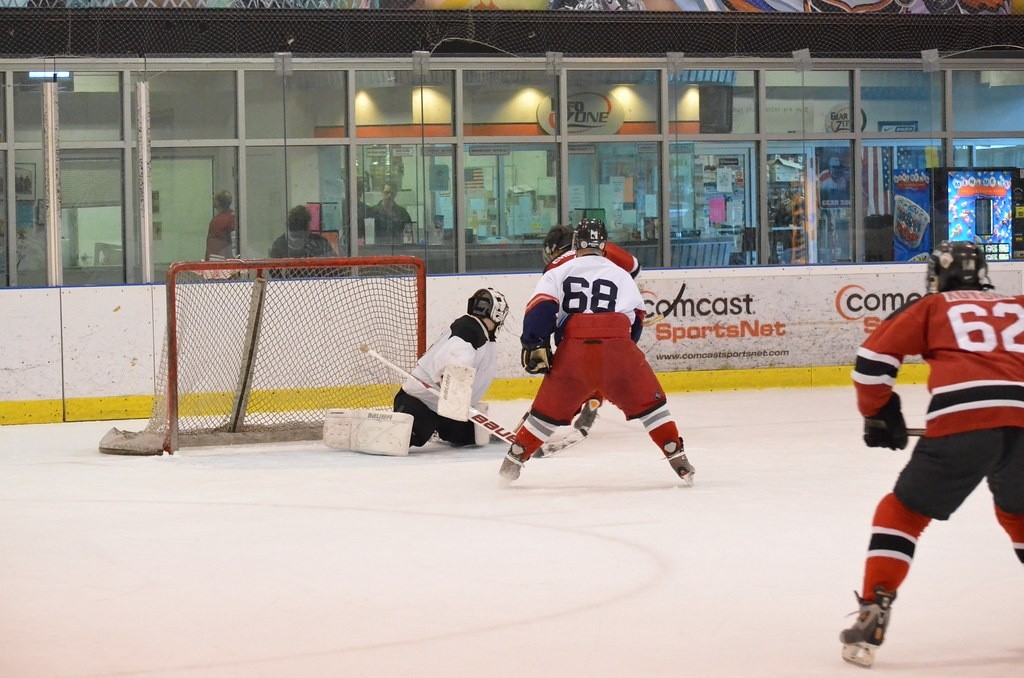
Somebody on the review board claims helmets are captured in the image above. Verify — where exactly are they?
[543,224,579,265]
[212,190,232,208]
[468,288,509,343]
[574,219,608,251]
[926,241,995,294]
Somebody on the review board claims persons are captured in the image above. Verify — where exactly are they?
[839,241,1023,667]
[342,181,372,240]
[542,224,640,431]
[498,218,696,486]
[269,205,343,278]
[323,288,508,457]
[370,181,411,244]
[206,189,236,262]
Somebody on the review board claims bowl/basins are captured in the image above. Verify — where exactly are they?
[894,195,930,248]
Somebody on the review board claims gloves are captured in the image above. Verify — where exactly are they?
[520,333,554,374]
[863,390,909,451]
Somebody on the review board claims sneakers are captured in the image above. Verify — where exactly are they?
[840,587,897,667]
[662,437,696,487]
[499,443,527,480]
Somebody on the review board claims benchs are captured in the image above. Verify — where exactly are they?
[358,233,737,288]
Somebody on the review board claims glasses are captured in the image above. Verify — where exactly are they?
[382,191,397,197]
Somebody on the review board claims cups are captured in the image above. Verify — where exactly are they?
[403,222,412,243]
[433,214,445,229]
[364,217,374,244]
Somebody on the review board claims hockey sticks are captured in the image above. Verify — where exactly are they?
[527,282,687,369]
[360,343,591,458]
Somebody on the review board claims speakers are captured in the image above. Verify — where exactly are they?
[698,82,733,134]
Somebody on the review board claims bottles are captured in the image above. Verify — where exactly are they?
[471,210,479,227]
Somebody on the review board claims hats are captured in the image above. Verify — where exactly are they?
[829,157,841,167]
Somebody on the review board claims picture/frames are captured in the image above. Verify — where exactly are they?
[0,162,36,201]
